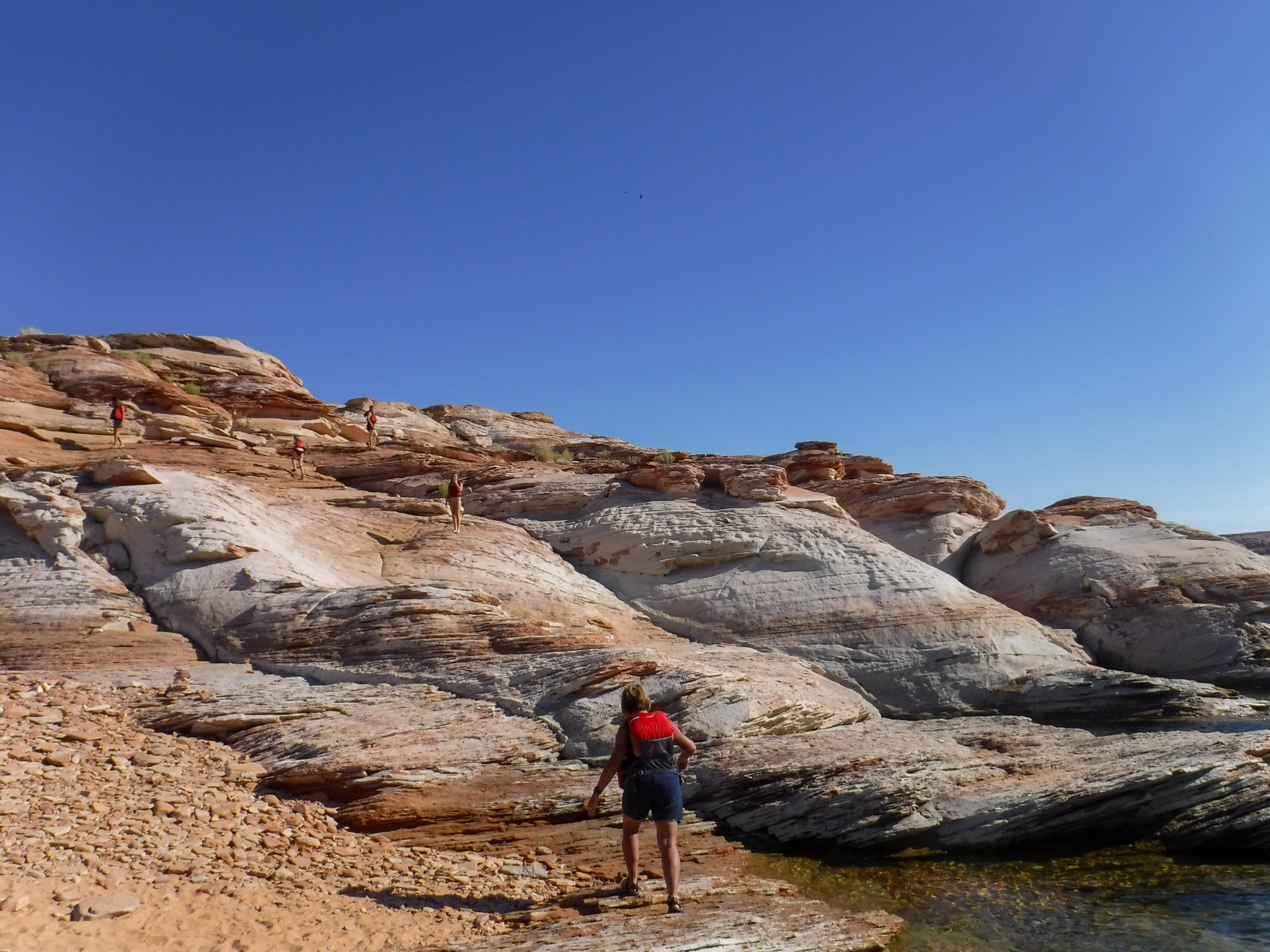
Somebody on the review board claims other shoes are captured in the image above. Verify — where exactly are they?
[621,876,639,895]
[668,900,683,913]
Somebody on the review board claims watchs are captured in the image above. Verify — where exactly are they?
[593,786,602,795]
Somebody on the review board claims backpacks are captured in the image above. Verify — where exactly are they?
[627,710,674,758]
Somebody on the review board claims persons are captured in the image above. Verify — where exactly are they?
[291,435,306,480]
[587,684,696,913]
[445,472,463,534]
[109,396,124,449]
[364,406,378,447]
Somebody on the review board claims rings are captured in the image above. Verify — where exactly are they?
[589,807,593,810]
